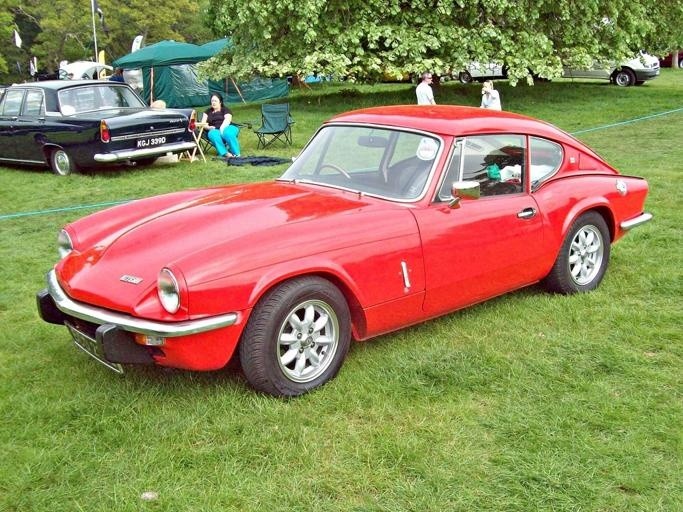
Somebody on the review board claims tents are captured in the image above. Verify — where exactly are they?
[107,38,289,110]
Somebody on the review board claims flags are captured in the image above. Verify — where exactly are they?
[93,0,109,39]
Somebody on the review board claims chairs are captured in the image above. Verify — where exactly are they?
[177,102,296,163]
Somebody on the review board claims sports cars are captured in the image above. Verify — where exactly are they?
[34,102,655,401]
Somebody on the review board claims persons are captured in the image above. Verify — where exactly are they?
[414,71,436,105]
[200,94,240,161]
[477,79,501,111]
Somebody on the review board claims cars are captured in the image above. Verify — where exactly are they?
[0,78,198,176]
